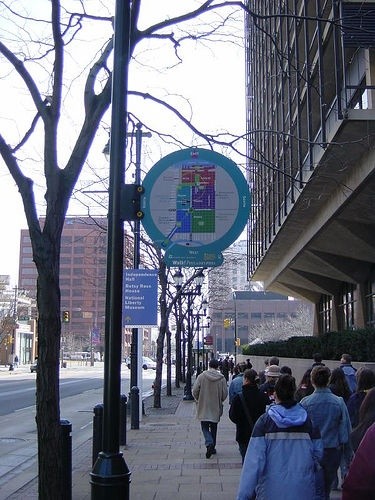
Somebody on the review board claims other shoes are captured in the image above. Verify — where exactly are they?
[206,443,216,459]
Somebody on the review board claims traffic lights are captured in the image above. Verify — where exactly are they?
[62,311,70,323]
[223,318,231,328]
[235,338,240,346]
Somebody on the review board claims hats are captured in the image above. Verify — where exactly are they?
[264,365,282,376]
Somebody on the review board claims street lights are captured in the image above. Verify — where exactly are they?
[172,269,205,401]
[199,316,212,372]
[190,299,208,378]
[9,284,26,371]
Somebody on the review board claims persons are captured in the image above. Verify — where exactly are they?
[192,353,375,500]
[15,356,18,368]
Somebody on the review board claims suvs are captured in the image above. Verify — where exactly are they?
[126,355,156,371]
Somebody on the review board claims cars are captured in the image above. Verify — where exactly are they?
[30,359,38,373]
